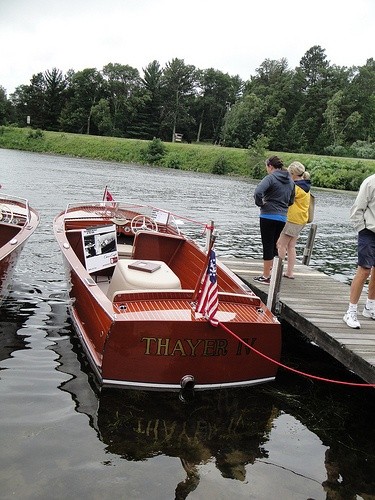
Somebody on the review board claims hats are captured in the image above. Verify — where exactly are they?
[287,161,305,175]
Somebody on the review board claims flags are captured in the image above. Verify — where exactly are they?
[195,243,219,327]
[106,191,115,206]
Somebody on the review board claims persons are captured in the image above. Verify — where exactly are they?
[276,161,312,279]
[252,154,297,285]
[342,173,375,329]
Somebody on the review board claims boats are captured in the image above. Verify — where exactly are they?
[0,184,41,299]
[52,185,283,394]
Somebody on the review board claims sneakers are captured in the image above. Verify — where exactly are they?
[343,310,361,329]
[361,307,375,319]
[252,275,270,284]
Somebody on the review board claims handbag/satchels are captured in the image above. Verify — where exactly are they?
[308,194,315,223]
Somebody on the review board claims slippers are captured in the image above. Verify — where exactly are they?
[283,273,295,279]
